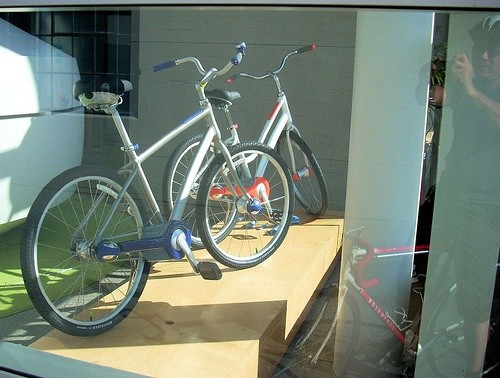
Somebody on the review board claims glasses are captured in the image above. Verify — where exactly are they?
[473,43,498,56]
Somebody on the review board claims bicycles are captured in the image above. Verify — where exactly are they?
[259,226,499,378]
[20,42,295,336]
[162,43,329,249]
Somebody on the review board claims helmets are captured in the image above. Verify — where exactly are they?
[469,14,500,45]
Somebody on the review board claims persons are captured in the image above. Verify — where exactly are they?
[378,14,499,376]
